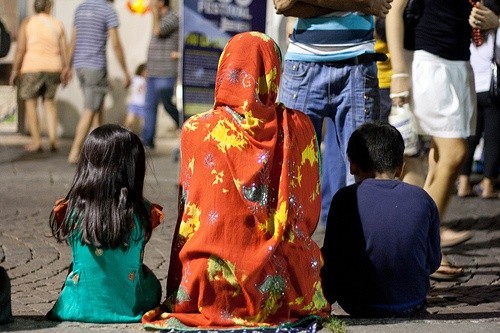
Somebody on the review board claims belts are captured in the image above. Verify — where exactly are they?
[316,53,388,69]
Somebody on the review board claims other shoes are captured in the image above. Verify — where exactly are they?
[439,225,474,248]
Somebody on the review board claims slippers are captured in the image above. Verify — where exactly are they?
[429,259,458,279]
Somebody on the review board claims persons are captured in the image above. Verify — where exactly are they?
[272,0,500,247]
[61,0,131,163]
[44,124,164,324]
[141,31,332,333]
[0,18,11,58]
[16,0,71,142]
[125,0,187,149]
[319,121,442,317]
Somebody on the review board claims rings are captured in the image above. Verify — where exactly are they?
[476,21,481,25]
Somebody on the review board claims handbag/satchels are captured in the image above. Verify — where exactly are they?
[388,102,420,155]
[374,0,425,45]
